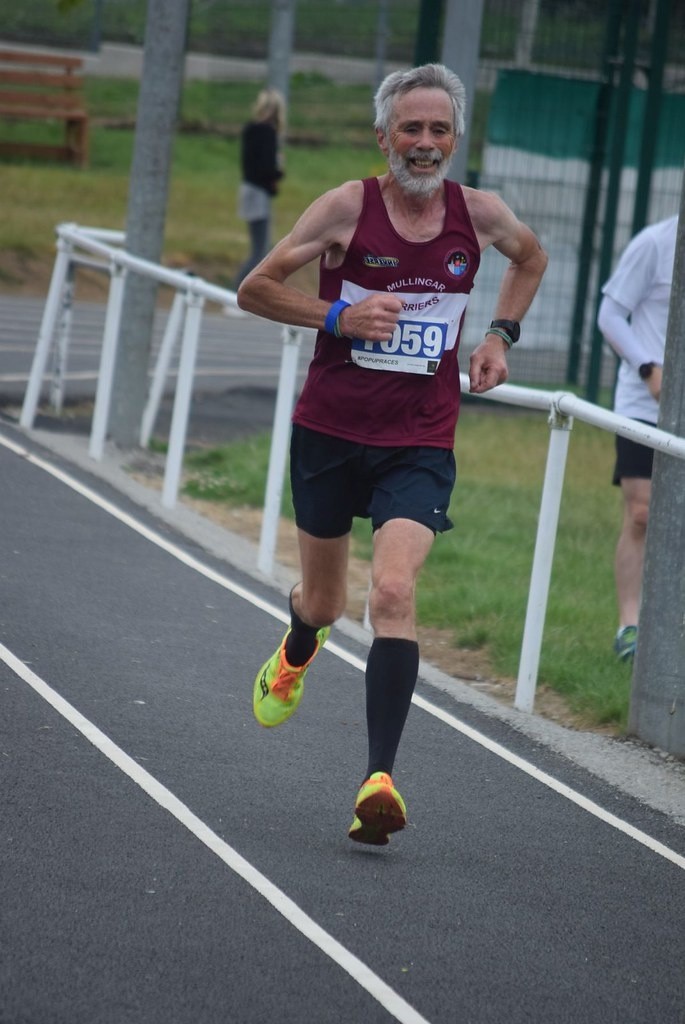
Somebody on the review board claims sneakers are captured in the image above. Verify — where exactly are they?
[613,626,638,661]
[347,771,406,845]
[254,626,330,728]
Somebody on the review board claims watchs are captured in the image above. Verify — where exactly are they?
[490,318,521,343]
[639,361,657,380]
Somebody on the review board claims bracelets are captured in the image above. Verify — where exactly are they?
[485,328,513,350]
[325,300,352,337]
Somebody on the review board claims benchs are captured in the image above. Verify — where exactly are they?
[0,51,89,170]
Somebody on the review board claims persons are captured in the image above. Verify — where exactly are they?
[597,214,680,675]
[238,62,549,846]
[235,88,287,285]
